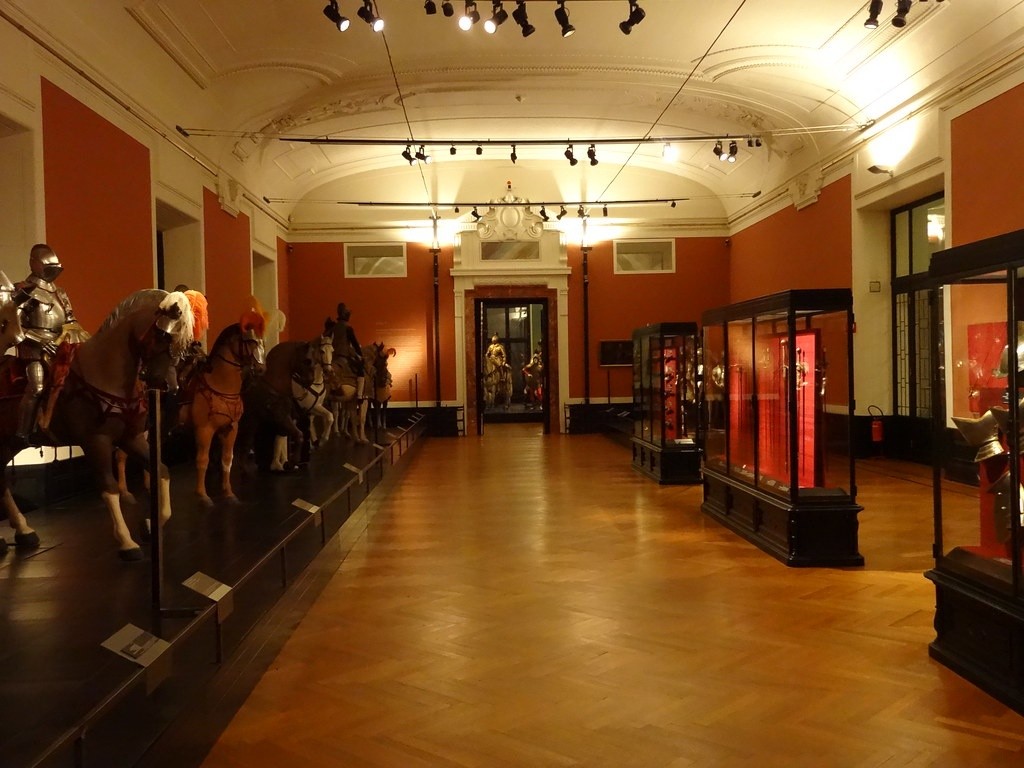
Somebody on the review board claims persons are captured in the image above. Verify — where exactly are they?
[522,353,544,411]
[326,302,366,403]
[14,244,76,445]
[485,332,507,381]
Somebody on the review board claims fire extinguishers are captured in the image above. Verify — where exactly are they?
[871,417,884,443]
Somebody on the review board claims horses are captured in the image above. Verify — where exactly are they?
[0,270,395,559]
